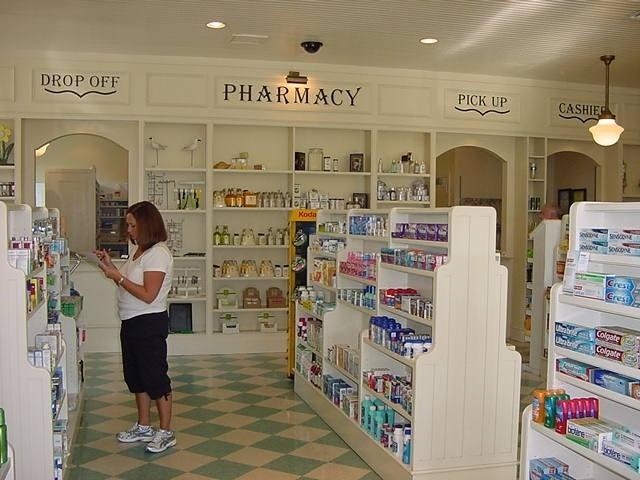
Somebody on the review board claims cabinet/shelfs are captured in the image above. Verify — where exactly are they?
[373,132,432,207]
[0,200,84,479]
[94,180,130,262]
[213,125,293,336]
[519,201,640,480]
[509,136,546,357]
[0,118,14,202]
[295,206,521,479]
[140,123,207,335]
[293,128,373,210]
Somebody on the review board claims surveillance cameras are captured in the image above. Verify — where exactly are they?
[301,41,324,54]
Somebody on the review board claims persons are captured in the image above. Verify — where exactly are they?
[95,201,176,453]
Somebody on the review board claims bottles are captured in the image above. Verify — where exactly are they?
[310,359,323,390]
[296,317,322,344]
[213,224,291,246]
[378,151,427,174]
[345,196,354,210]
[354,198,360,209]
[360,394,412,464]
[333,158,337,172]
[226,187,291,208]
[323,157,332,171]
[377,181,431,202]
[530,163,536,179]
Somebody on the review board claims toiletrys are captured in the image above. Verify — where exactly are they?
[369,316,432,361]
[377,152,430,201]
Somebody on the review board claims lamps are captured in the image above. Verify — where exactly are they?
[588,55,625,147]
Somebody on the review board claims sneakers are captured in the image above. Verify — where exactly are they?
[116,423,155,443]
[145,429,177,453]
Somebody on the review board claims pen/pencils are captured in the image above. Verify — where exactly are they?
[100,247,110,261]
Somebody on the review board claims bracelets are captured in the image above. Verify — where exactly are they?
[118,276,125,284]
